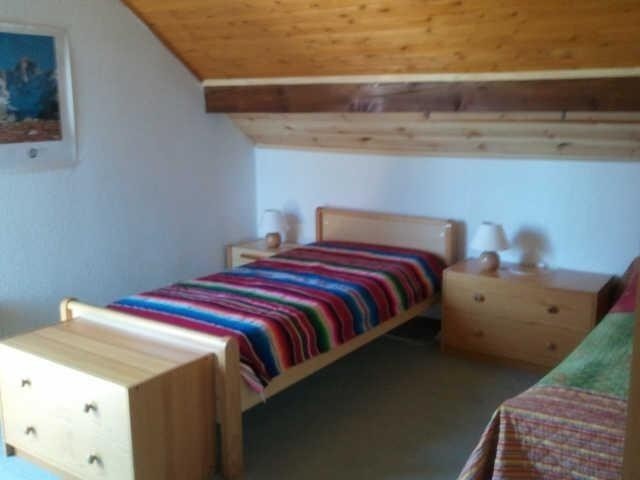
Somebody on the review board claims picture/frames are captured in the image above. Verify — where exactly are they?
[0,20,81,183]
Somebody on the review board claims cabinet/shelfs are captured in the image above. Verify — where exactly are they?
[0,319,224,480]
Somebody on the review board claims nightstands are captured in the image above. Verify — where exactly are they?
[439,255,619,371]
[223,235,299,280]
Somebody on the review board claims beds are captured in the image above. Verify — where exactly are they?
[493,236,638,480]
[52,202,461,478]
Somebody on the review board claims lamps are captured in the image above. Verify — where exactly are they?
[473,219,507,273]
[259,206,284,250]
